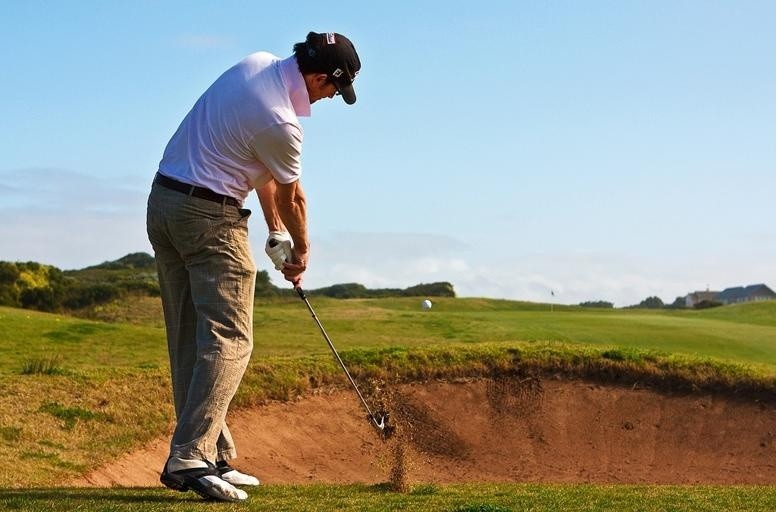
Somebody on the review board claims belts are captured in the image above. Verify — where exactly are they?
[154,170,242,209]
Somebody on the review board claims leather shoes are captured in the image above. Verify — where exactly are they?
[217,460,259,487]
[159,456,249,502]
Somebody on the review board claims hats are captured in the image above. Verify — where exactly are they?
[306,30,363,105]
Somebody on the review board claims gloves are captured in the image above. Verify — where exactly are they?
[265,231,293,270]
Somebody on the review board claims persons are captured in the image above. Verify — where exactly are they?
[147,31,360,502]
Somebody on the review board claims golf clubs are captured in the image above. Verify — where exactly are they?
[268,238,385,430]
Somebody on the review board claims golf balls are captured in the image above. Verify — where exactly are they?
[423,300,431,310]
